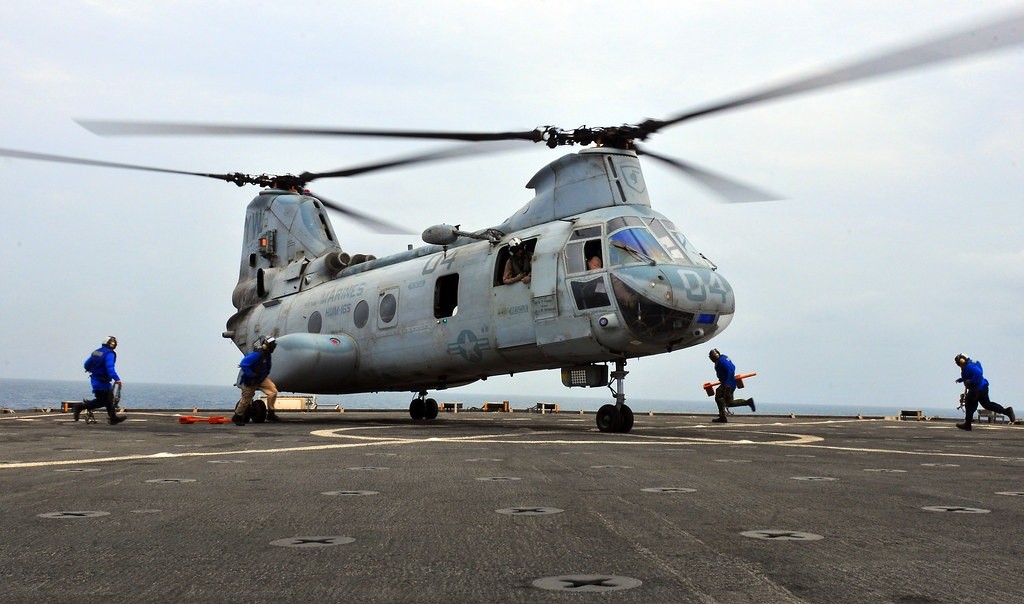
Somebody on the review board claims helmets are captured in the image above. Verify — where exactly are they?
[102,336,118,349]
[709,349,720,362]
[955,355,967,366]
[261,337,276,344]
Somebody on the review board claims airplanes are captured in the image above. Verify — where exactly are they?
[1,12,1023,425]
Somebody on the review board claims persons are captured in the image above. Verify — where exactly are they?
[951,354,1016,431]
[707,349,756,422]
[231,336,282,426]
[72,335,129,425]
[585,256,603,271]
[501,238,533,285]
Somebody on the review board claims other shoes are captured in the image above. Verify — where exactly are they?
[956,421,973,430]
[232,415,246,426]
[110,416,127,425]
[74,404,81,421]
[712,416,727,422]
[267,411,281,421]
[748,398,755,412]
[1005,406,1016,422]
